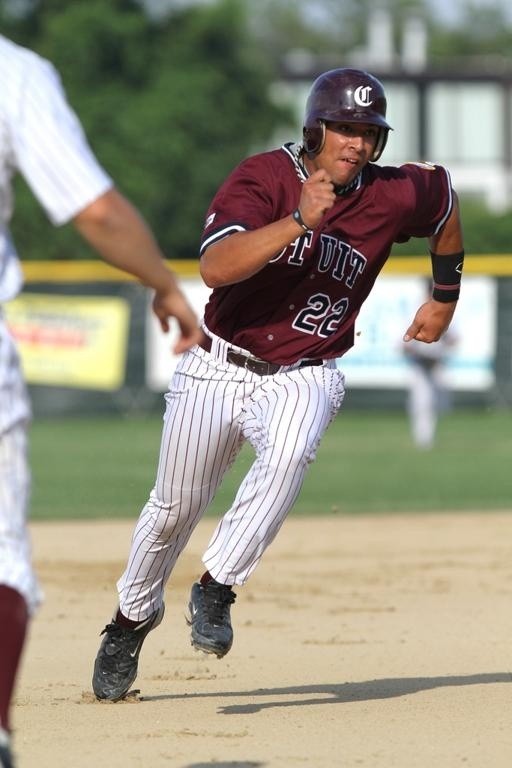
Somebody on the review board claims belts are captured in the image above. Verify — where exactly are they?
[198,326,323,376]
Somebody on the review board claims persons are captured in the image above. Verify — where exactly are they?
[0,34,204,767]
[91,57,467,701]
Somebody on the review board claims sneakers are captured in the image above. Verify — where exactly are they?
[185,579,236,659]
[93,601,165,700]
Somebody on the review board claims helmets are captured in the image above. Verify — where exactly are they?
[303,68,393,162]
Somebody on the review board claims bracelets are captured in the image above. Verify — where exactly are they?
[428,249,464,302]
[291,208,311,232]
